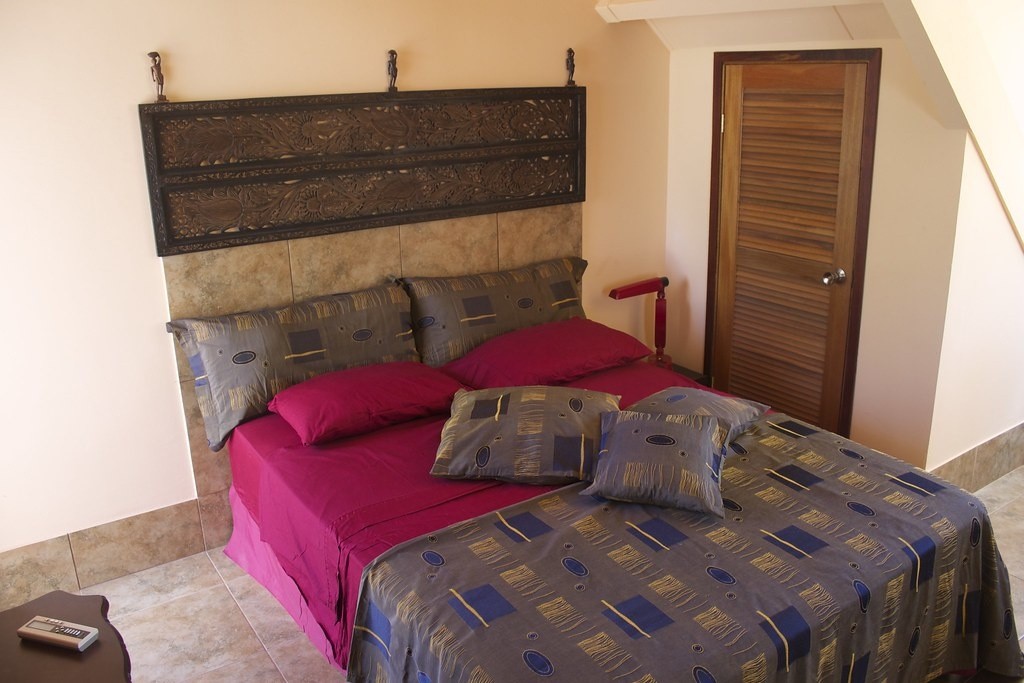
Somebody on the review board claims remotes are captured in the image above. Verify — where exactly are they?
[17,615,98,652]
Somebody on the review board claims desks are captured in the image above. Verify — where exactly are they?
[0,590,132,683]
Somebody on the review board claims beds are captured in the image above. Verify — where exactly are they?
[137,48,1024,683]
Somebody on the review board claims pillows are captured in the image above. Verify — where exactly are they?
[268,364,474,447]
[397,256,588,365]
[581,410,733,518]
[166,275,421,452]
[625,385,771,442]
[429,385,622,483]
[440,317,654,390]
